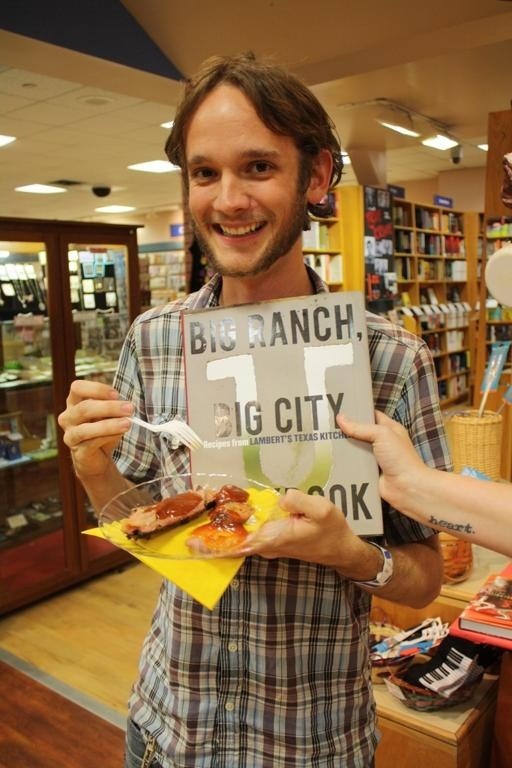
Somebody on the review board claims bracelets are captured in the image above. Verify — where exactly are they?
[352,541,393,592]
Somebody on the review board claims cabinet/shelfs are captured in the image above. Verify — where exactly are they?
[0,215,150,618]
[301,182,512,417]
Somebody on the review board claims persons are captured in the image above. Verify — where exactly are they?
[59,53,456,742]
[329,244,512,560]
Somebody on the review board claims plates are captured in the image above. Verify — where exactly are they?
[99,474,292,559]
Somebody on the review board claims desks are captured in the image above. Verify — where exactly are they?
[367,563,512,768]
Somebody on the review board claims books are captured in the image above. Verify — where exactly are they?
[395,204,512,409]
[181,293,383,540]
[458,575,510,637]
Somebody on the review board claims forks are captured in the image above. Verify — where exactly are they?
[123,412,204,451]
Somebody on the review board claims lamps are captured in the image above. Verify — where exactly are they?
[374,105,421,140]
[417,125,461,153]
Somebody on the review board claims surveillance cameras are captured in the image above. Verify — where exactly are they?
[93,188,111,197]
[450,146,463,164]
